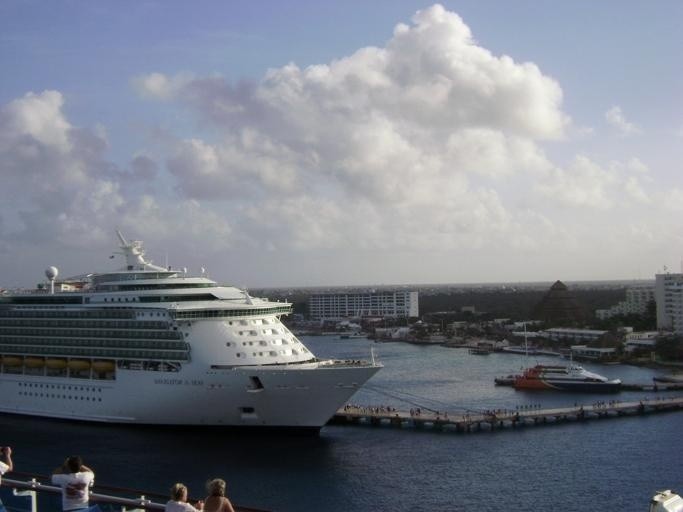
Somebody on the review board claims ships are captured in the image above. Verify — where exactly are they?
[0,230,385,436]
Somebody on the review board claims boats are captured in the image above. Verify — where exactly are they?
[653,373,683,390]
[494,321,621,392]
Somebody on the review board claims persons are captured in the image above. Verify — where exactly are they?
[164,482,204,512]
[203,478,234,512]
[0,446,12,511]
[50,454,94,511]
